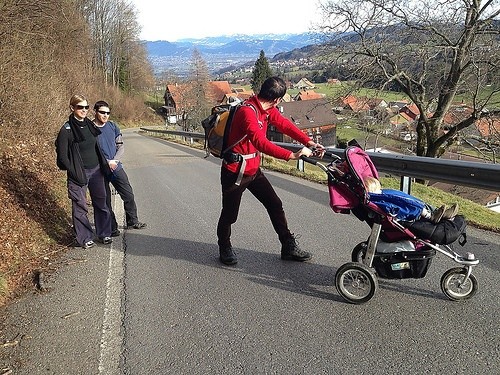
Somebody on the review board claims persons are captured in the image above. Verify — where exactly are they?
[362,175,459,223]
[55,94,113,249]
[91,100,146,237]
[217,76,325,265]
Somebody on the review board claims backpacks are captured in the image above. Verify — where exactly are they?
[201,92,258,159]
[54,122,71,170]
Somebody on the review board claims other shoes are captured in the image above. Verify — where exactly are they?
[112,228,120,236]
[84,240,96,248]
[127,221,147,229]
[97,236,113,244]
[444,204,459,220]
[429,205,446,225]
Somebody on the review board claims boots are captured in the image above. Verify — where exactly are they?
[278,236,314,260]
[218,239,238,264]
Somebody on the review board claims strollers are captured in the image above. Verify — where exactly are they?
[298,144,480,305]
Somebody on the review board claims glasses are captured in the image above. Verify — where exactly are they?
[77,105,89,109]
[98,111,111,115]
